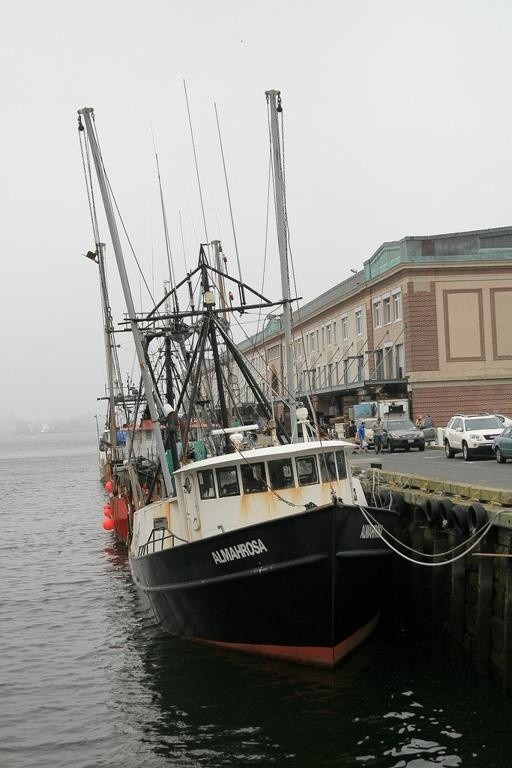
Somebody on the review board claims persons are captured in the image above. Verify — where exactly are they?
[370,416,385,454]
[347,418,357,452]
[358,421,370,452]
[424,413,434,448]
[415,414,424,428]
[116,423,130,445]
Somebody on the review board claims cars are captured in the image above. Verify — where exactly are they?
[355,411,512,464]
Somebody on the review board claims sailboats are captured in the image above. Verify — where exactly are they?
[74,75,397,669]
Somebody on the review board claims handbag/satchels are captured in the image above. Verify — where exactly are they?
[362,439,369,447]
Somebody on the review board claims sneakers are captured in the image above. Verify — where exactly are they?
[359,442,431,456]
